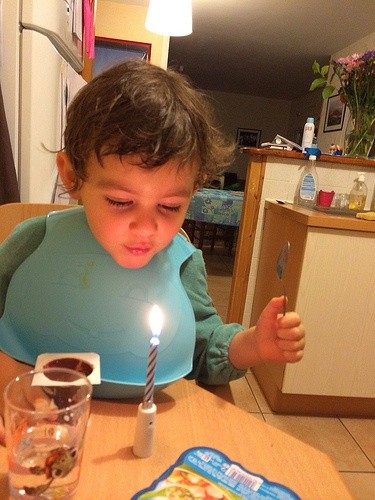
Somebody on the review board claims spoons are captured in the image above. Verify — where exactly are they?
[275,241,291,315]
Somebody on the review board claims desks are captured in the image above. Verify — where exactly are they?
[185,188,245,256]
[0,348,355,500]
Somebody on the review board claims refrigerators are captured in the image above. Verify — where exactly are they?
[1,0,89,204]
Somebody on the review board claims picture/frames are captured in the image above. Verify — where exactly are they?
[236,128,262,148]
[323,94,347,132]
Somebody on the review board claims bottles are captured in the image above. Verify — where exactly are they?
[302,117,315,153]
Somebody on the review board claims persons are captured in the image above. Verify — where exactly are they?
[0,59,306,448]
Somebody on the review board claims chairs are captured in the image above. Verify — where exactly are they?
[190,176,225,251]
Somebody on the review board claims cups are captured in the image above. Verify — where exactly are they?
[317,190,335,207]
[3,367,93,500]
[336,193,349,211]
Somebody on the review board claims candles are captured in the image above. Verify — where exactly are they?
[143,304,165,407]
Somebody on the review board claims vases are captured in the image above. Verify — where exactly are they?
[343,133,375,158]
[309,49,375,134]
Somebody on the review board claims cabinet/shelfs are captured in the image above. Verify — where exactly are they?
[227,146,375,419]
